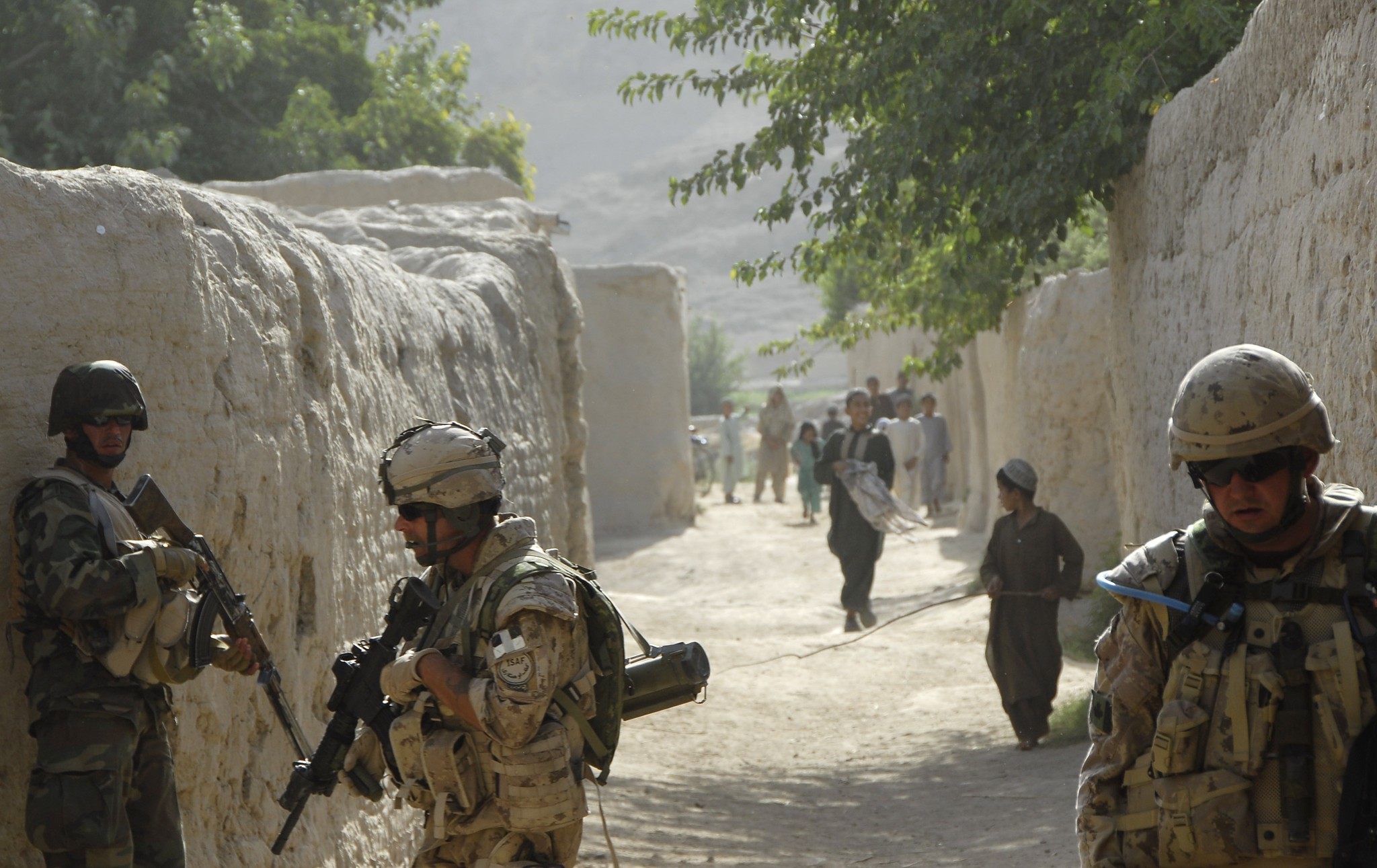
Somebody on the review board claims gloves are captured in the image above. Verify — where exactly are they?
[379,648,441,706]
[337,725,386,800]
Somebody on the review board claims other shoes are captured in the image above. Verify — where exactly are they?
[1019,717,1051,750]
[845,598,875,632]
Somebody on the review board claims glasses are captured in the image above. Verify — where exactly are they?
[83,412,135,426]
[398,504,423,522]
[1188,449,1287,487]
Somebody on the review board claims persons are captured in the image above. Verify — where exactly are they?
[1075,341,1376,868]
[716,371,953,526]
[814,387,895,633]
[14,359,262,868]
[338,421,599,868]
[979,458,1086,751]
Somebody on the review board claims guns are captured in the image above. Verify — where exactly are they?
[271,577,442,856]
[122,473,313,762]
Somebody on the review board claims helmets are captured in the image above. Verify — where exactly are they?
[379,422,507,509]
[1168,344,1341,471]
[47,360,148,436]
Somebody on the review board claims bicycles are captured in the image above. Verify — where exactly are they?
[690,426,715,498]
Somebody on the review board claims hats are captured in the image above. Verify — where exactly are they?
[895,393,912,408]
[845,387,872,403]
[1002,458,1038,492]
